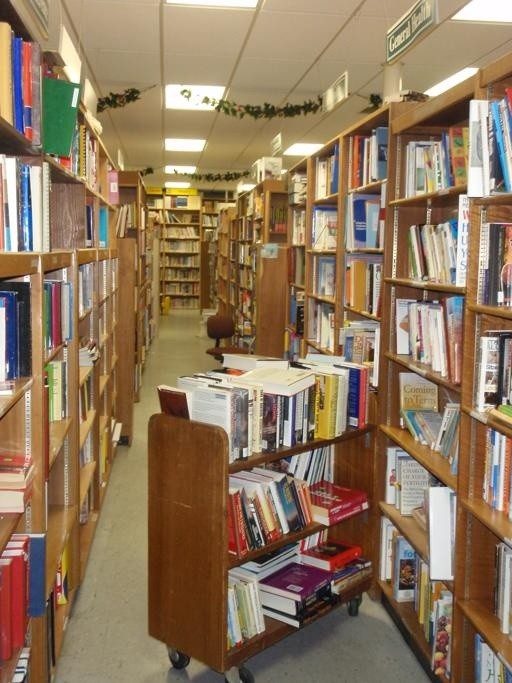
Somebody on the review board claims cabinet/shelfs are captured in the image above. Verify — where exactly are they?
[1,0,511,682]
